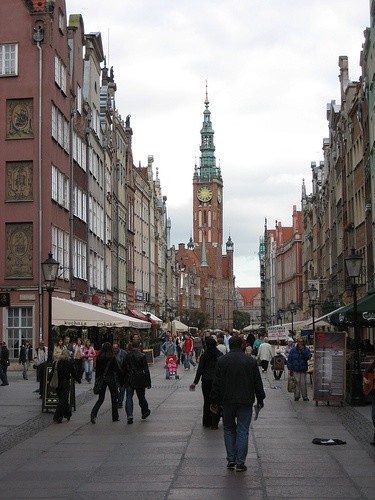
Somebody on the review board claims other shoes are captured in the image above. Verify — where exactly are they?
[170,372,172,374]
[173,372,175,374]
[91,413,96,424]
[227,461,236,468]
[127,419,133,424]
[236,464,247,471]
[113,419,119,422]
[141,409,151,421]
[0,383,8,386]
[89,379,92,384]
[295,398,299,401]
[304,397,309,401]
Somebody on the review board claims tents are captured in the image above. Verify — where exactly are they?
[164,319,189,331]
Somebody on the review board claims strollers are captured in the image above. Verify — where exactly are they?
[164,353,180,380]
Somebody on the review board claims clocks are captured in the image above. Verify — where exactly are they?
[197,186,213,202]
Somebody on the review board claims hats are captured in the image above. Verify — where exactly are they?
[243,335,247,340]
[205,331,211,336]
[217,338,224,343]
[169,358,173,362]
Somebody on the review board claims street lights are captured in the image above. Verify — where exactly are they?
[277,310,285,326]
[39,250,60,398]
[307,283,319,332]
[344,247,369,407]
[166,307,174,339]
[288,299,297,339]
[186,316,190,332]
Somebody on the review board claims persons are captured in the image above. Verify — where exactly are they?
[53,338,69,364]
[343,337,375,354]
[286,340,310,402]
[366,359,375,446]
[32,340,49,382]
[19,339,32,380]
[50,349,79,424]
[0,341,9,386]
[58,324,275,473]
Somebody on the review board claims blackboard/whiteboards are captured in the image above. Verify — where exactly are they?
[143,349,154,365]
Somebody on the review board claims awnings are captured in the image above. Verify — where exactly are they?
[245,291,375,334]
[50,297,153,332]
[129,309,164,328]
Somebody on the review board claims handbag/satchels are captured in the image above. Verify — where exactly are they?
[49,359,58,394]
[94,376,104,394]
[288,375,297,392]
